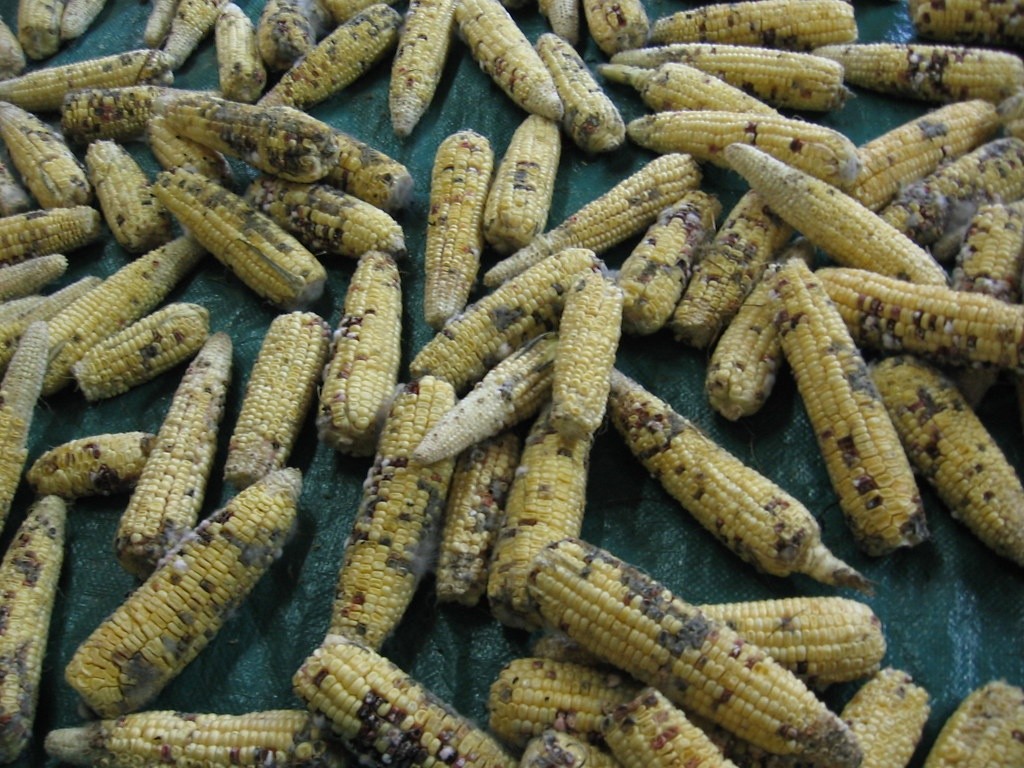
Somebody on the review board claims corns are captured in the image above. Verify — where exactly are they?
[0,0,1024,768]
